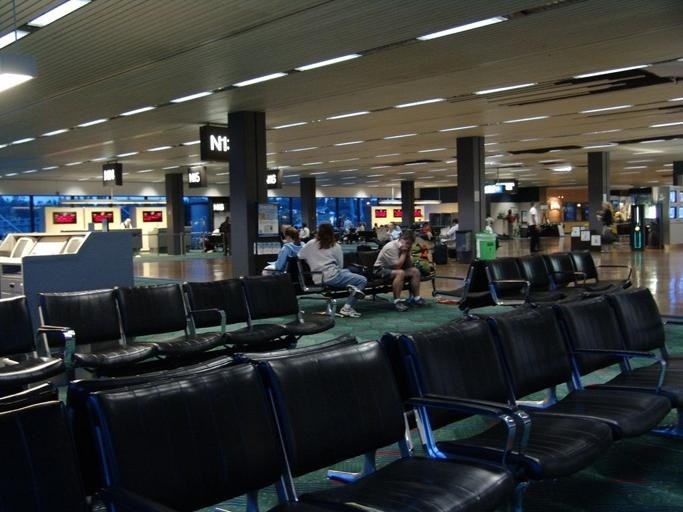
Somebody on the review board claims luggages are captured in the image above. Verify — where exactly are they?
[431,236,448,265]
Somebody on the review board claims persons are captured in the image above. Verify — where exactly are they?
[259,207,460,317]
[221,216,232,256]
[202,226,221,253]
[504,209,516,236]
[118,219,132,230]
[527,200,545,252]
[595,200,614,252]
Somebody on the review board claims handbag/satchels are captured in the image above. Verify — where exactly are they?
[410,254,431,275]
[350,263,373,280]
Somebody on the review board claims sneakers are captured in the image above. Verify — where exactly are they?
[391,299,408,312]
[346,285,365,299]
[339,307,362,318]
[410,296,426,305]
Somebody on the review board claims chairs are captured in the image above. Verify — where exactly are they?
[0,247,683,510]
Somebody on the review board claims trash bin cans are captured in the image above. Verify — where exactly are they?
[475,233,497,261]
